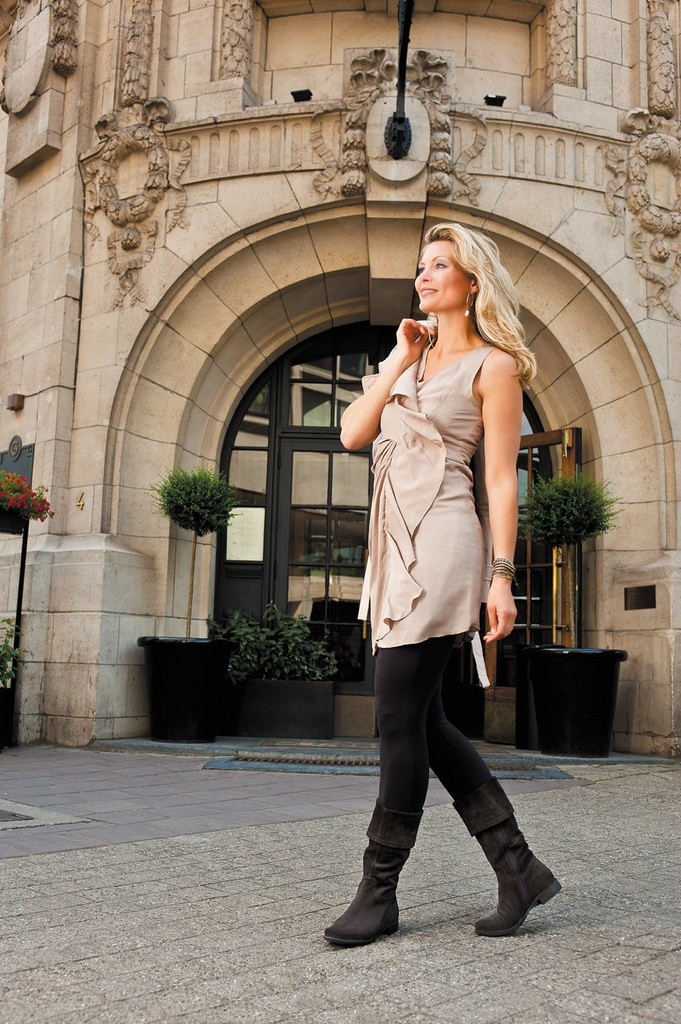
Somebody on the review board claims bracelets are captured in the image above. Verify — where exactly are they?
[491,558,516,580]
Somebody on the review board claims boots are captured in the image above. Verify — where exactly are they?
[324,797,424,945]
[452,775,562,937]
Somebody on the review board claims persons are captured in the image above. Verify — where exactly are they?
[323,222,561,946]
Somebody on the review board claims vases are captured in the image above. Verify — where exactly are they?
[0,505,24,534]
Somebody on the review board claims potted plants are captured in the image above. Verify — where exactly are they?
[208,603,338,740]
[135,459,246,744]
[0,618,28,750]
[513,462,629,757]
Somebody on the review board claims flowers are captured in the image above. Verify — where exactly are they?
[0,469,56,523]
